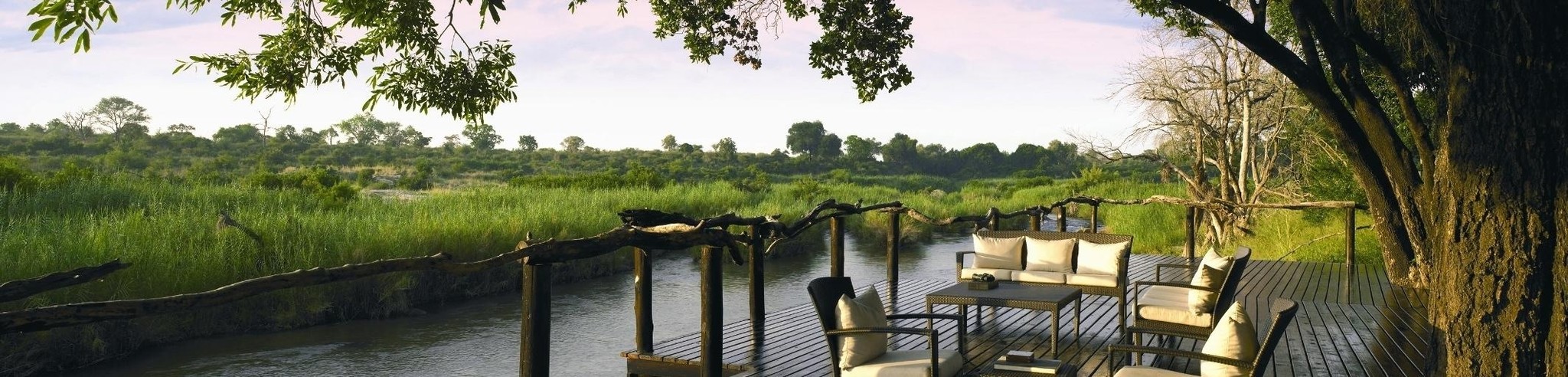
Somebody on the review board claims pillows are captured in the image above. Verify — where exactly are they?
[834,284,888,370]
[1188,247,1233,317]
[1076,240,1128,278]
[1024,236,1076,275]
[1200,300,1261,376]
[972,233,1025,271]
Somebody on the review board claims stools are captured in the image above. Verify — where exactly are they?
[969,357,1077,377]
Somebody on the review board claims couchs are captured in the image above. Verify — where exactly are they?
[957,231,1134,345]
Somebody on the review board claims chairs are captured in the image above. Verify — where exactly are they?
[1106,297,1299,376]
[808,277,967,376]
[1134,245,1251,366]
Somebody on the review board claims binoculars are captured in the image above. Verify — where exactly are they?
[972,273,995,282]
